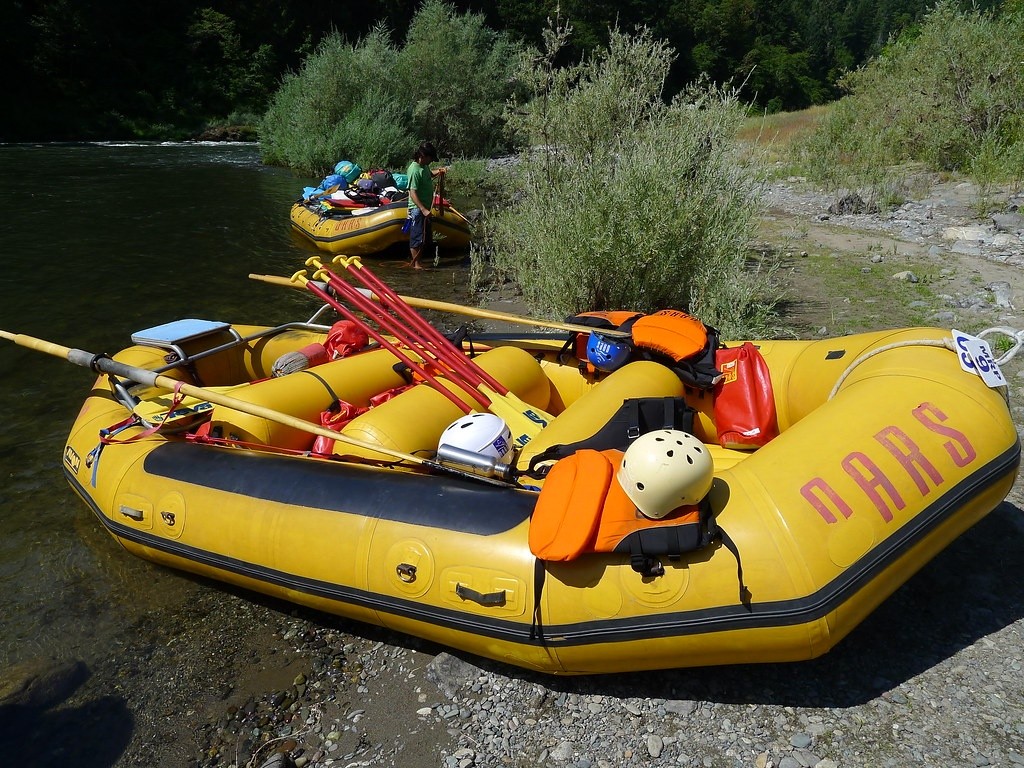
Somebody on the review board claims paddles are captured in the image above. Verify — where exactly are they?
[1,253,636,491]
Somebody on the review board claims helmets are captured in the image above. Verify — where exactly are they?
[586,330,632,372]
[617,429,715,520]
[436,412,514,479]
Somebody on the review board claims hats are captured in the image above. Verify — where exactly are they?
[420,143,440,162]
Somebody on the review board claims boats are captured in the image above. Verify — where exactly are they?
[62,308,1024,678]
[289,179,470,257]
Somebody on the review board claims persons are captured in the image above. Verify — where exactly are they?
[406,142,447,273]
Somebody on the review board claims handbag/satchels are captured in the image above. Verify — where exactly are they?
[715,342,781,449]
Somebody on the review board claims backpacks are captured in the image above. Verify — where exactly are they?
[320,160,408,202]
